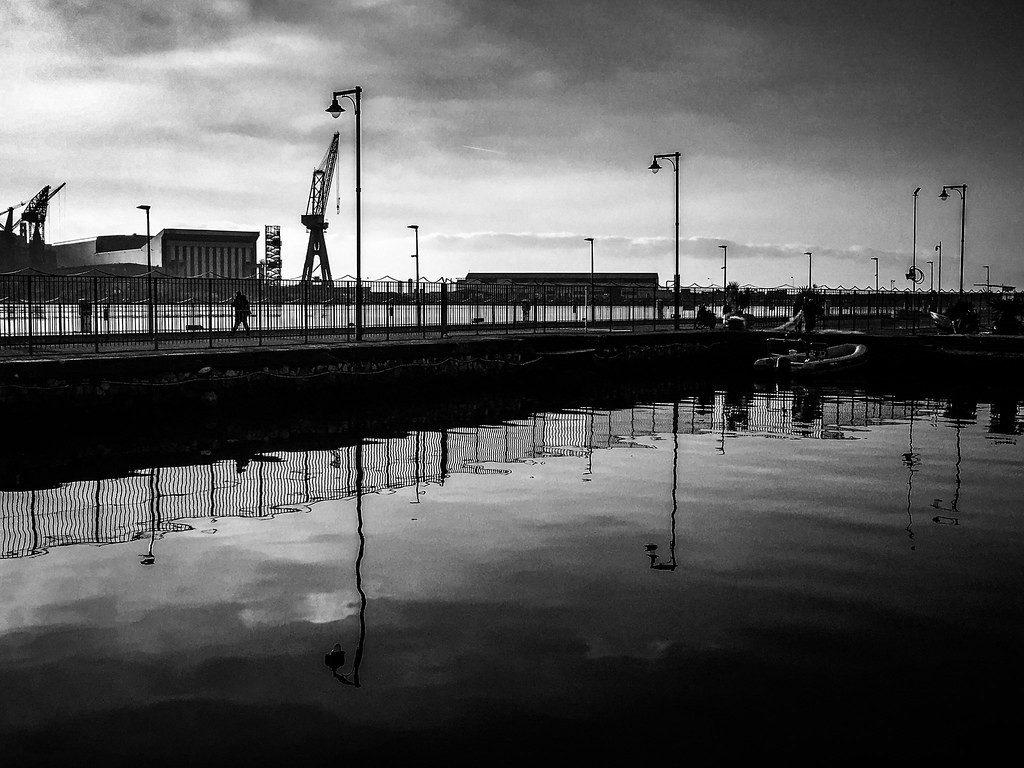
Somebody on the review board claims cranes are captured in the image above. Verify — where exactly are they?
[295,129,341,306]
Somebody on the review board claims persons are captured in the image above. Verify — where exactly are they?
[231,291,250,331]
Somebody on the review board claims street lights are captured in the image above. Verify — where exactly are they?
[938,183,968,335]
[407,225,421,333]
[325,85,363,342]
[982,265,990,325]
[648,151,682,331]
[871,257,879,319]
[584,238,595,328]
[718,245,728,327]
[136,204,154,339]
[804,252,813,291]
[927,261,934,333]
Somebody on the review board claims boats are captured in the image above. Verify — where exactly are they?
[752,333,873,388]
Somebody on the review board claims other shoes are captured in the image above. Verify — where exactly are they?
[246,327,250,336]
[226,332,233,336]
